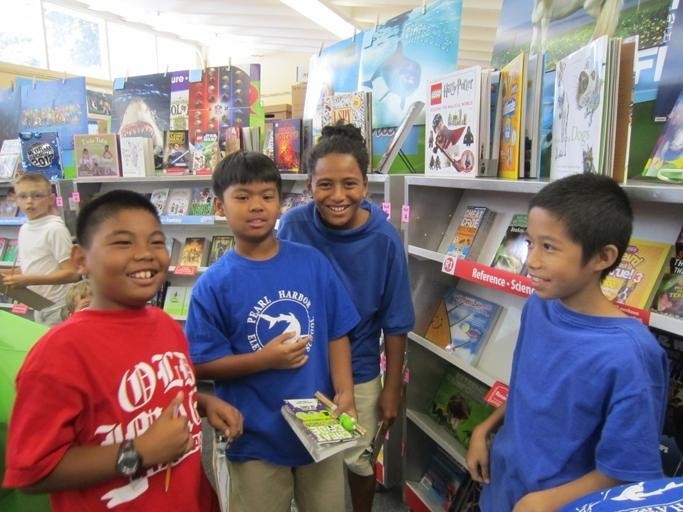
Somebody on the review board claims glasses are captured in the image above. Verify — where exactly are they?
[14,194,51,199]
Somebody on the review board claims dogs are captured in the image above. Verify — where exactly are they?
[574,48,603,127]
[581,147,596,175]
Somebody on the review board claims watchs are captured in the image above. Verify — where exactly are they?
[116,437,143,481]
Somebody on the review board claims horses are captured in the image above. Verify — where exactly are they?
[528,0,624,59]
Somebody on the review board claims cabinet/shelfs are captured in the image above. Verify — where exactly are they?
[71,174,403,394]
[402,175,683,512]
[0,180,73,310]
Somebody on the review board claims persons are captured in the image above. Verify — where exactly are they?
[2,189,245,511]
[185,152,362,511]
[465,173,669,511]
[277,119,415,511]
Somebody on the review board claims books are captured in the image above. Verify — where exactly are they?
[651,228,683,319]
[446,206,497,262]
[281,399,371,463]
[426,34,683,182]
[73,133,121,180]
[119,134,155,178]
[146,279,194,321]
[1,189,26,218]
[647,327,683,448]
[601,239,675,310]
[166,235,235,267]
[490,214,530,275]
[427,367,504,453]
[374,100,426,175]
[151,184,216,216]
[279,189,312,218]
[424,287,504,366]
[420,445,482,511]
[1,236,21,262]
[163,89,372,175]
[0,131,65,181]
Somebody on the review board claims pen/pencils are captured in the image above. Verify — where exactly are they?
[315,392,368,437]
[4,248,18,294]
[165,404,178,493]
